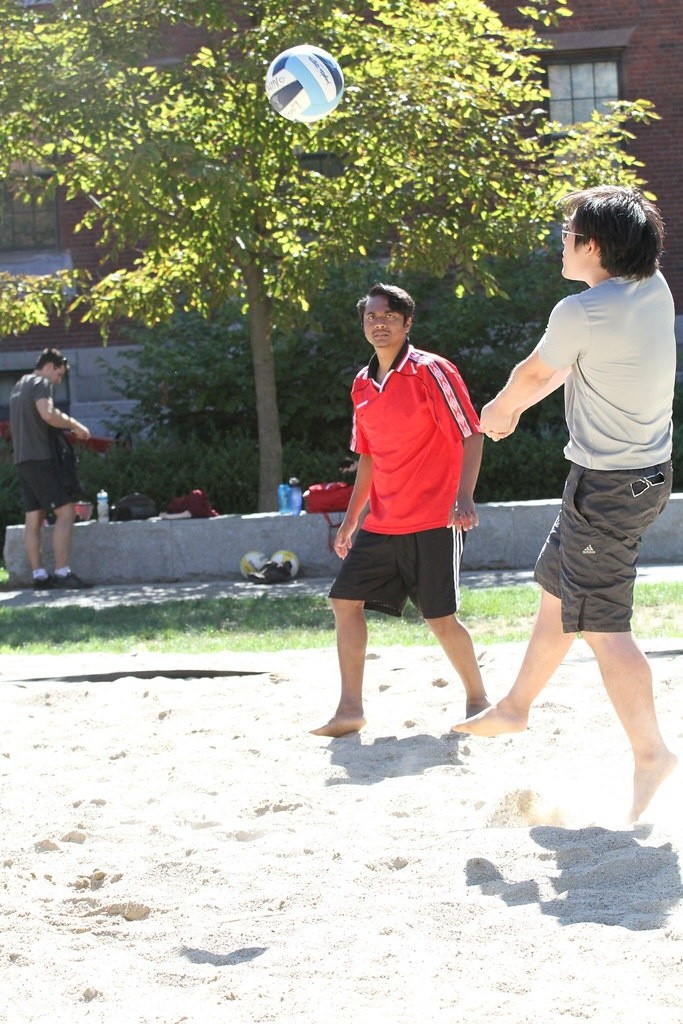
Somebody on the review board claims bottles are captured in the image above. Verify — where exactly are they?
[97,489,109,524]
[289,477,302,515]
[277,484,288,514]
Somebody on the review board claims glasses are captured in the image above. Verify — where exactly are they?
[562,225,584,242]
[62,357,67,364]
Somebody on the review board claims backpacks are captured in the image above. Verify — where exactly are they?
[109,494,156,521]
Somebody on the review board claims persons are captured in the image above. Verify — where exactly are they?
[310,284,491,738]
[452,191,678,823]
[10,348,94,588]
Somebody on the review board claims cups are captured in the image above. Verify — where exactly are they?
[72,504,94,521]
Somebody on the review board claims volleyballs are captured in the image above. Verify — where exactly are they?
[272,549,300,580]
[239,550,269,579]
[265,45,345,124]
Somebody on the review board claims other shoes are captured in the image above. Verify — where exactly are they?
[52,572,91,589]
[34,577,54,590]
[250,560,293,583]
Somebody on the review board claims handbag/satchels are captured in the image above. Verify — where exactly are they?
[159,490,219,518]
[304,480,355,528]
